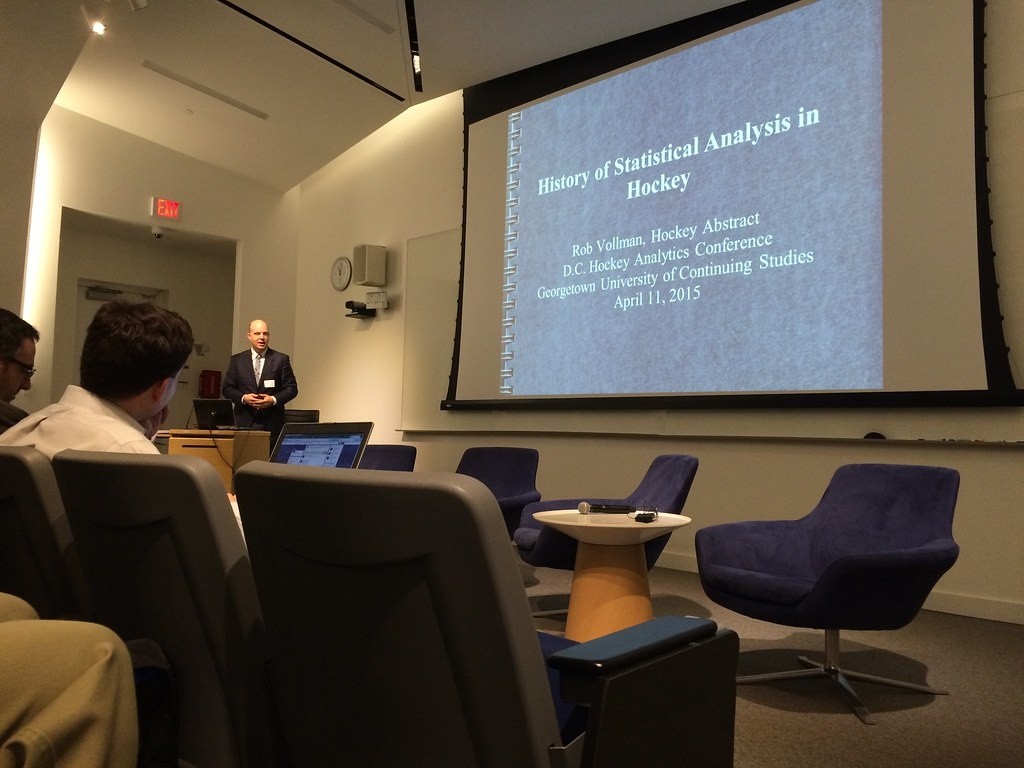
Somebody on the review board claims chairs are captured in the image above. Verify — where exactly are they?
[0,443,738,768]
[696,462,961,727]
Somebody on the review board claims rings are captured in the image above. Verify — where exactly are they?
[257,407,259,410]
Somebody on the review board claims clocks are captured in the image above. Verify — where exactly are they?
[331,257,352,292]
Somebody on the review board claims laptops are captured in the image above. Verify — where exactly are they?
[193,398,253,430]
[268,421,375,469]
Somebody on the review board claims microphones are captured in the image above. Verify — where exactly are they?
[578,501,636,514]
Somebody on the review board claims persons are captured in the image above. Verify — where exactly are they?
[0,590,141,768]
[0,298,199,462]
[221,319,300,456]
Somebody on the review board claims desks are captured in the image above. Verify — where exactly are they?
[531,510,692,644]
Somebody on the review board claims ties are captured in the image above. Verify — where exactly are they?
[254,354,262,386]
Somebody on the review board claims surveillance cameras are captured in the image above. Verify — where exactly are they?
[152,226,165,239]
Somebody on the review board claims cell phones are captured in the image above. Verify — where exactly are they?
[635,513,654,522]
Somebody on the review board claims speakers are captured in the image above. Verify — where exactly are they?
[353,244,386,286]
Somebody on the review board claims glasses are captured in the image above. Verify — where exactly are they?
[0,355,36,380]
